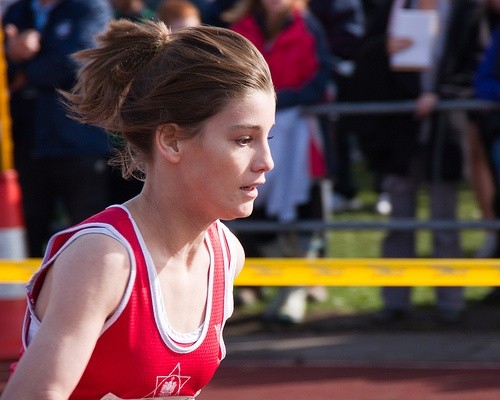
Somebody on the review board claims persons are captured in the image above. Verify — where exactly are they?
[0,0,500,324]
[0,17,276,400]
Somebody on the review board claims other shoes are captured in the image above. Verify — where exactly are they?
[371,307,411,323]
[440,311,466,332]
[235,286,329,331]
